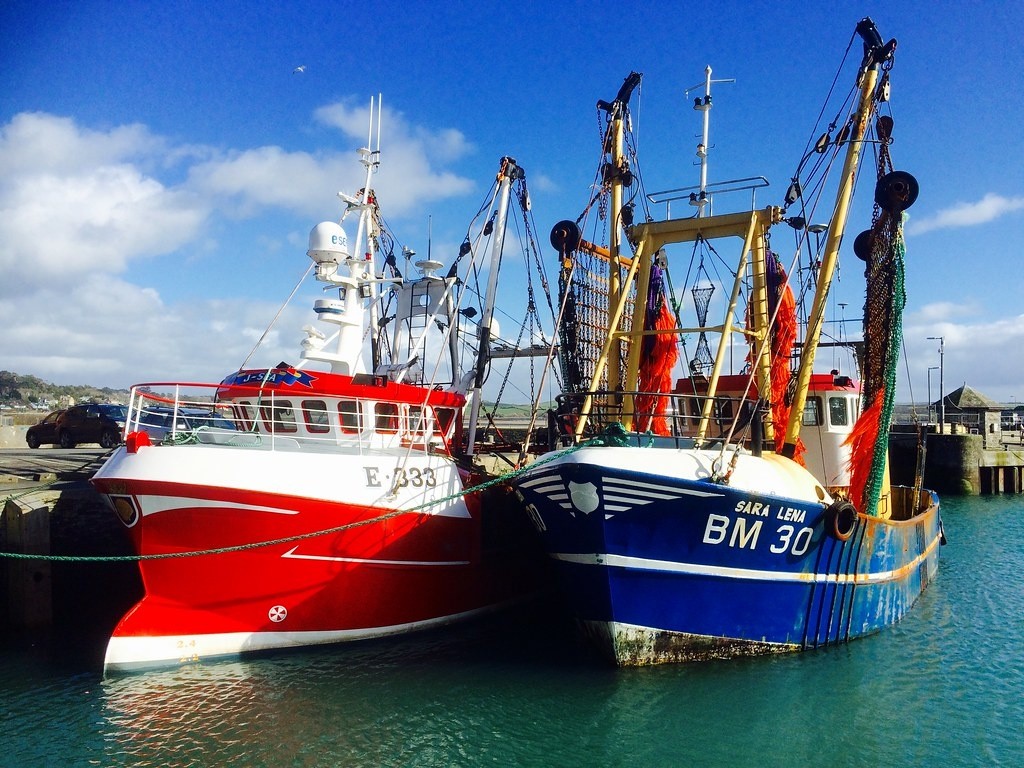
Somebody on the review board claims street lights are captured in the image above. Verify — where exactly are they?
[927,368,939,422]
[1010,396,1016,409]
[927,337,945,434]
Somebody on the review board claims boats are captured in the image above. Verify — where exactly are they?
[508,15,948,670]
[85,91,559,681]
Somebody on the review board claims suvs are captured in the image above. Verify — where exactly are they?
[121,406,241,447]
[55,403,136,448]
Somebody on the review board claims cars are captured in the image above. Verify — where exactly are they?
[26,409,66,449]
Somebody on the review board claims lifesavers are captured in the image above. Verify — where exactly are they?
[826,500,860,544]
[875,170,919,211]
[550,220,584,253]
